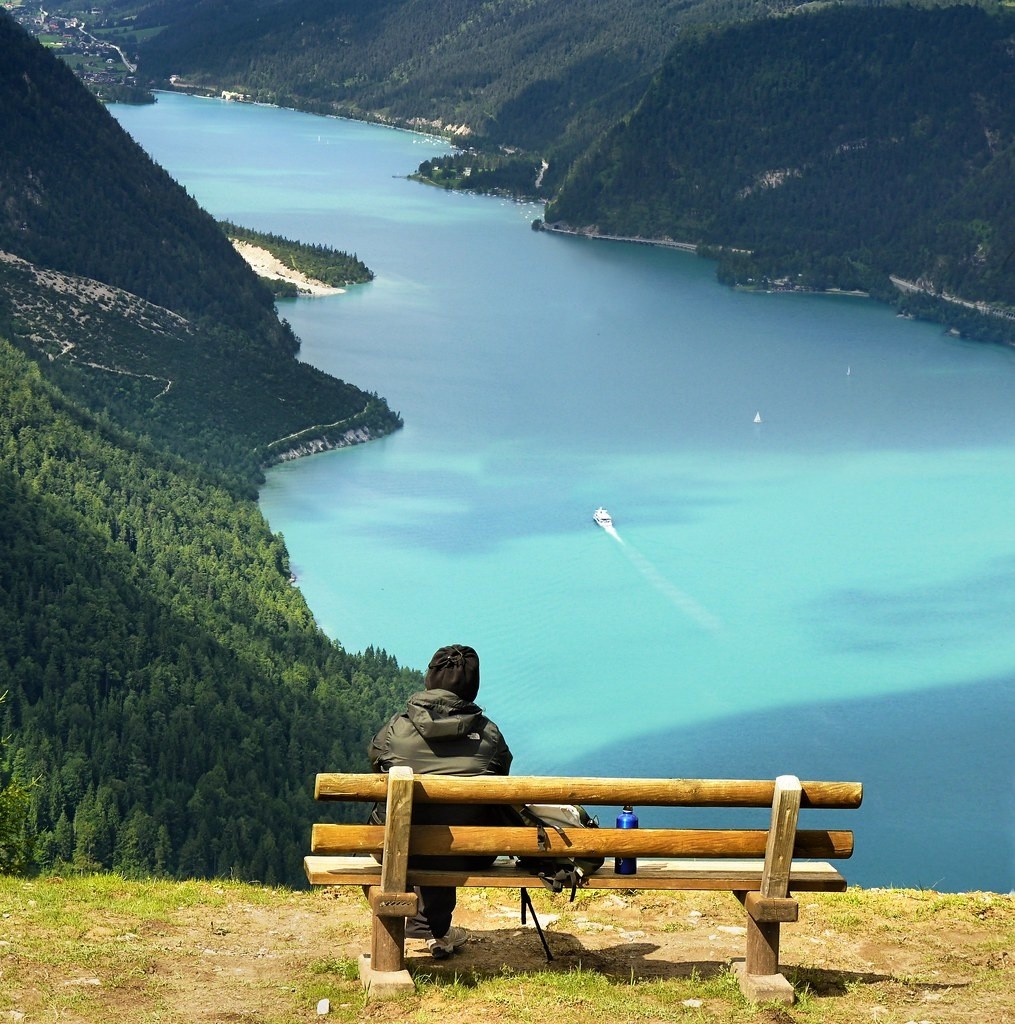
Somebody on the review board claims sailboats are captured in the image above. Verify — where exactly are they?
[754,412,764,424]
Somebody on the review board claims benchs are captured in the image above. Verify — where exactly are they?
[303,767,863,1003]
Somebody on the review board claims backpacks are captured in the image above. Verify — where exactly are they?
[514,804,605,902]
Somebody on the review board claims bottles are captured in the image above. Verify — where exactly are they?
[614,806,639,875]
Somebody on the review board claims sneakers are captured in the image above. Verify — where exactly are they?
[405,937,433,957]
[426,925,468,959]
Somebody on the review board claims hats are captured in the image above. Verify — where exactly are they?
[425,645,480,704]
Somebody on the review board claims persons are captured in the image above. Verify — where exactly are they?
[362,643,512,962]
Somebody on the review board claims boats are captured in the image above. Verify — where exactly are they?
[592,507,613,526]
